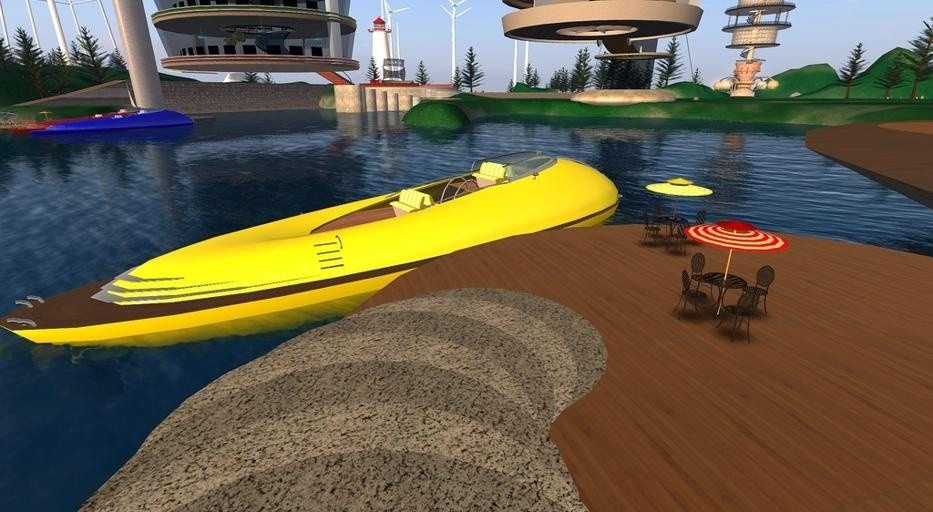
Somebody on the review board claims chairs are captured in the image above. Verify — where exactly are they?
[674,252,774,344]
[640,204,708,255]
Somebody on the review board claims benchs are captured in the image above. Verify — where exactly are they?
[388,189,435,218]
[470,161,506,189]
